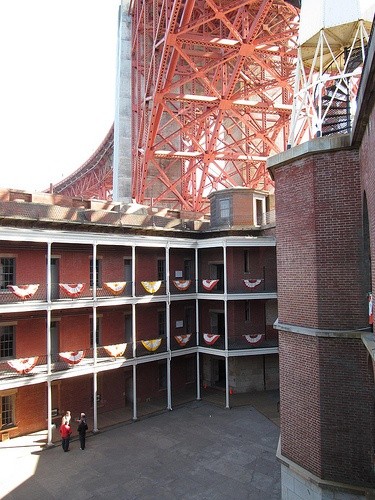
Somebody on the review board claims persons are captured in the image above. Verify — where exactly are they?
[59,420,72,452]
[75,412,87,424]
[77,414,89,452]
[61,410,72,426]
